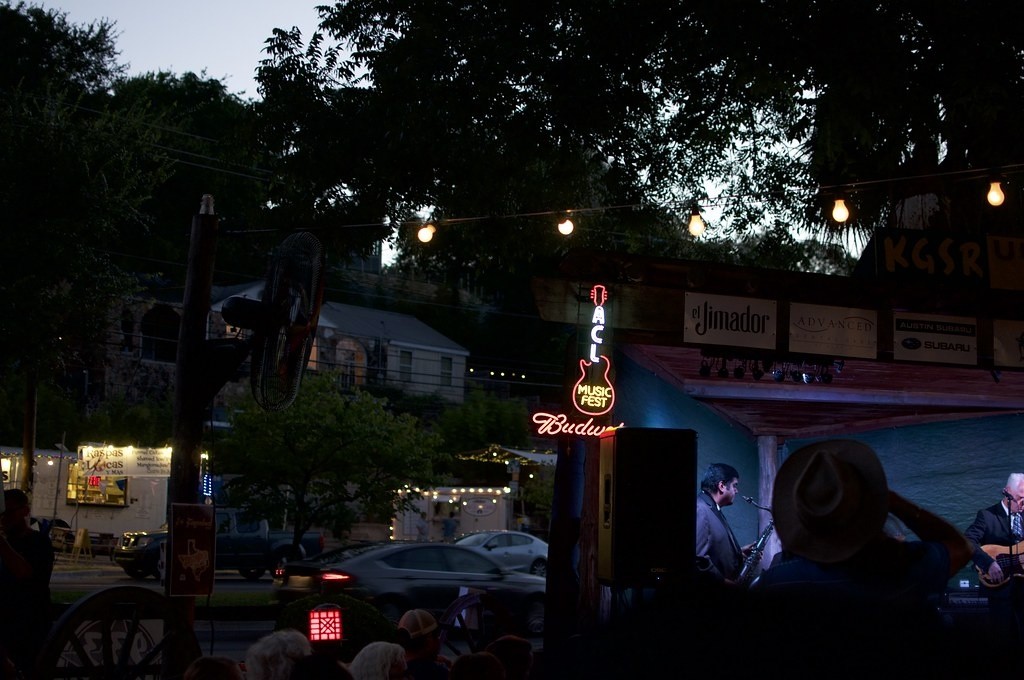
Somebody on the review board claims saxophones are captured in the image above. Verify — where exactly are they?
[736,494,774,586]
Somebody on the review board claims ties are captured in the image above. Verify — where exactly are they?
[1013,513,1024,542]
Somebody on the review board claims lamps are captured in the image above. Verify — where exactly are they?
[698,356,845,385]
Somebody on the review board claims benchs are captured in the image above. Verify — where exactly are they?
[64,541,113,550]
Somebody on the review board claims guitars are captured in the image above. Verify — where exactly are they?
[975,539,1024,588]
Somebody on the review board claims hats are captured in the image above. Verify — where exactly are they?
[772,438,888,564]
[398,609,438,637]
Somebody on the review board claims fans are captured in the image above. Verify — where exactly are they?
[202,229,325,422]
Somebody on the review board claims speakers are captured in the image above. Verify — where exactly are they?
[597,427,697,585]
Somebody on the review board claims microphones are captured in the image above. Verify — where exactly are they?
[1001,488,1017,502]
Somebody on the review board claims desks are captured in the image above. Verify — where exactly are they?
[54,526,114,561]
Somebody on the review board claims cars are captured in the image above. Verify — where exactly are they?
[283,539,549,638]
[449,530,548,577]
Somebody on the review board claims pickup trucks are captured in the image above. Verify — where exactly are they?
[112,507,325,581]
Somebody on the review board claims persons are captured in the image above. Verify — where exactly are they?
[762,439,973,680]
[962,471,1023,630]
[697,462,766,606]
[11,486,1023,680]
